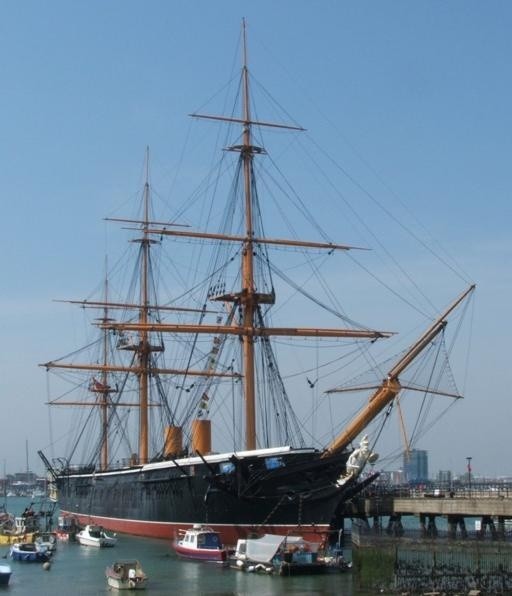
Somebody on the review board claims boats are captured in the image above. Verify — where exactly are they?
[0,500,119,584]
[172,524,353,576]
[104,559,149,590]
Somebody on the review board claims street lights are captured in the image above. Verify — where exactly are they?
[464,456,472,488]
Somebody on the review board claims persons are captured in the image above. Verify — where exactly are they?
[337,435,379,487]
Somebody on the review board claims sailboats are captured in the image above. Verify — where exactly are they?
[37,15,472,553]
